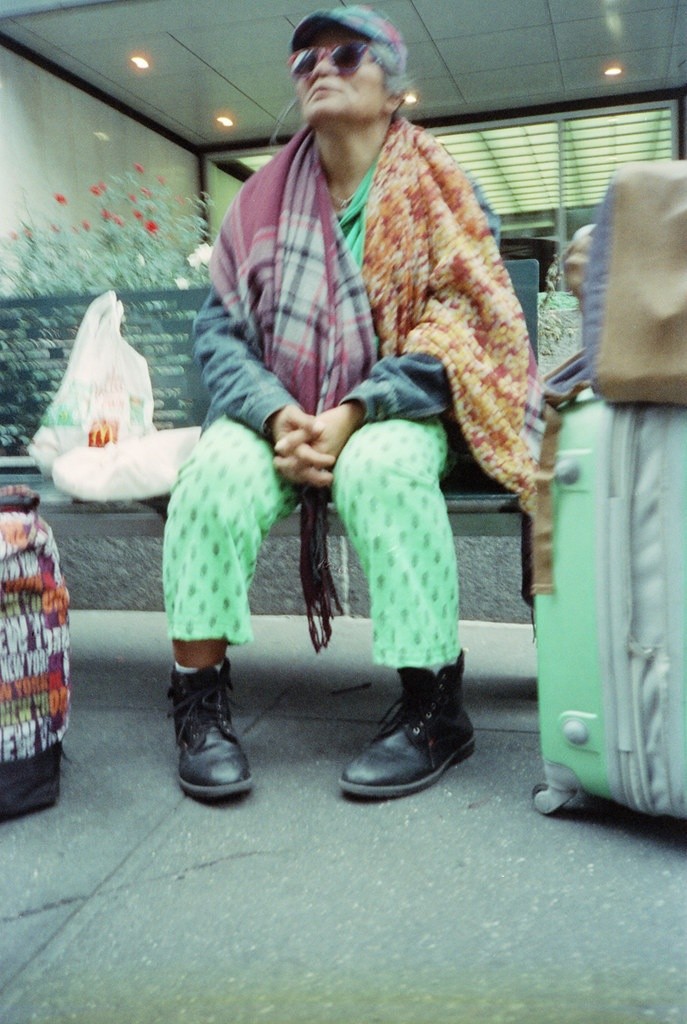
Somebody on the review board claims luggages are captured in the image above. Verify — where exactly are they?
[524,390,686,826]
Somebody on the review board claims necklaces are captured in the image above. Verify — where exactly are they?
[331,193,355,208]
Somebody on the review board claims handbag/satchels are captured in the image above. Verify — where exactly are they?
[524,159,687,409]
[0,484,74,822]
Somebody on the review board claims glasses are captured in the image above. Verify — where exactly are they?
[287,40,392,77]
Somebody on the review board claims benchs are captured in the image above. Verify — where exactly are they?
[0,257,540,607]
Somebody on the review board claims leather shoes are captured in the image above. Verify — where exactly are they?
[166,654,255,800]
[338,648,476,799]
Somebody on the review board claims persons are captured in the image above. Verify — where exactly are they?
[563,223,595,309]
[162,5,540,799]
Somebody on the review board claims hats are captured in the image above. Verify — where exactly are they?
[288,3,408,80]
